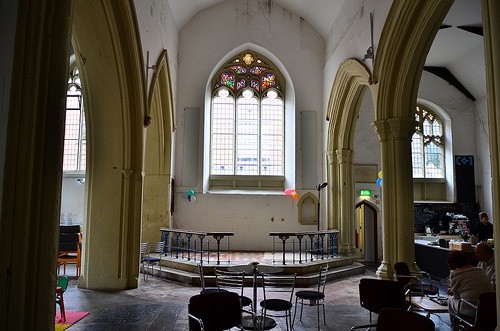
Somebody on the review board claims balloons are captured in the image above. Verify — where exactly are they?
[380,180,382,186]
[182,189,197,201]
[378,171,382,178]
[376,178,382,186]
[285,189,295,195]
[292,192,299,199]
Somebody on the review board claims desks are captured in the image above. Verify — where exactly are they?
[414,239,494,282]
[406,295,455,320]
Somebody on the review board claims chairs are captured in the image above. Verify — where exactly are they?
[291,262,332,331]
[188,292,260,331]
[349,261,497,331]
[55,275,69,318]
[140,240,166,283]
[57,224,83,279]
[260,271,298,331]
[140,241,149,282]
[214,268,256,331]
[197,264,229,294]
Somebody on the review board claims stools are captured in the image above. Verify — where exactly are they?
[56,287,66,322]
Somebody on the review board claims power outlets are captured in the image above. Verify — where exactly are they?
[281,217,284,221]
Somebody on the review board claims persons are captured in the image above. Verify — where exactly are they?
[446,250,491,323]
[471,212,493,241]
[475,241,495,291]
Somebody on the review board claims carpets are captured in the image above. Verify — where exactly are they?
[55,310,91,331]
